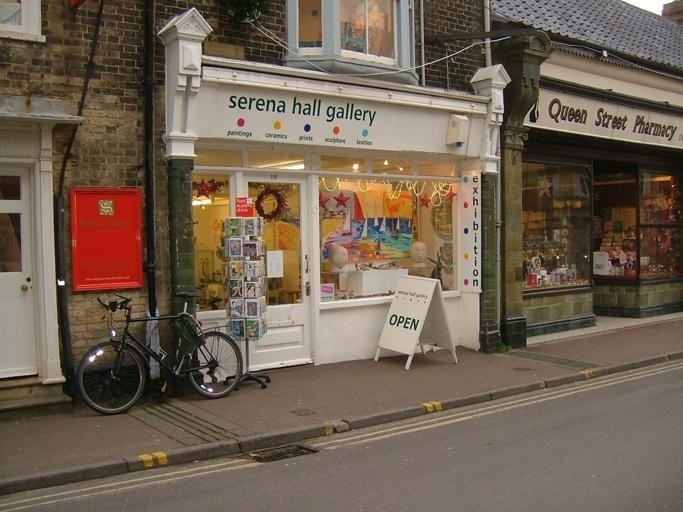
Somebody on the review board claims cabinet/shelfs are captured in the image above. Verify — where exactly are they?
[224,216,271,390]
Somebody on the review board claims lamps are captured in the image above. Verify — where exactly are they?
[192,196,215,205]
[258,159,304,169]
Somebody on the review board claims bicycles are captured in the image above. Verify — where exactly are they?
[77,291,241,414]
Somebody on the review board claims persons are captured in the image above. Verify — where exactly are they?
[625,225,635,240]
[328,244,349,273]
[230,217,259,338]
[410,240,427,267]
